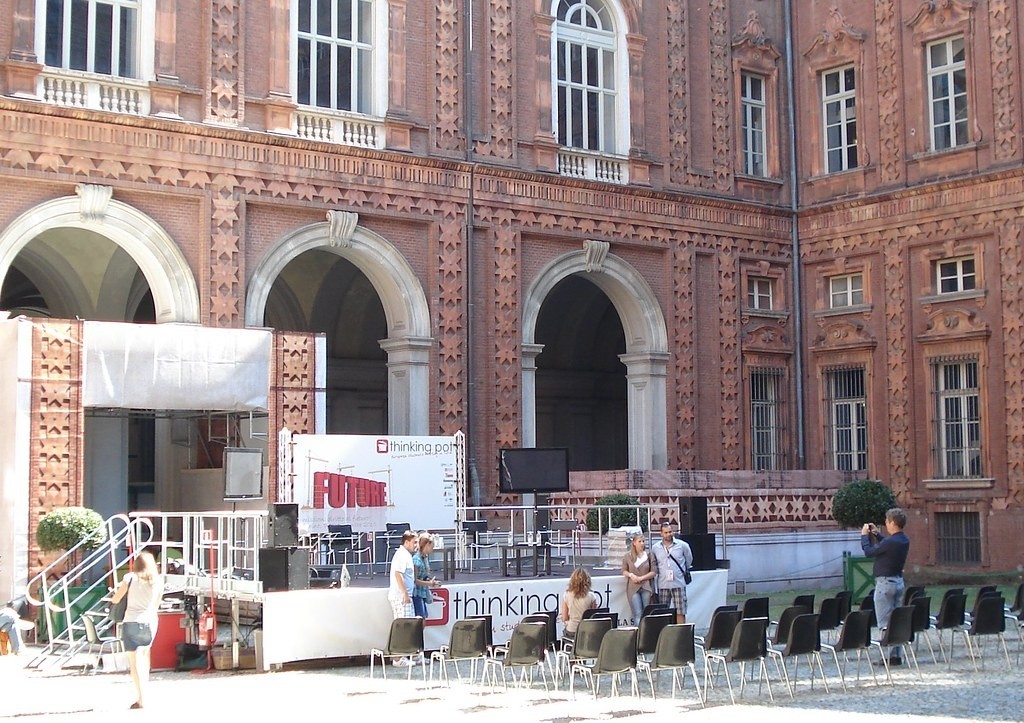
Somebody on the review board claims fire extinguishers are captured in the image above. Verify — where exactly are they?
[195,603,213,651]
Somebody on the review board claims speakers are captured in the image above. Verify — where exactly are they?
[258,547,310,593]
[679,496,708,535]
[675,532,716,570]
[268,503,299,548]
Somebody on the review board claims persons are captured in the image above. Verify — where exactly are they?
[652,521,694,624]
[860,508,910,666]
[623,533,657,654]
[559,567,597,674]
[0,608,35,659]
[110,552,163,709]
[388,533,418,668]
[409,533,442,665]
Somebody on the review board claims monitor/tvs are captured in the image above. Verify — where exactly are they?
[499,447,569,494]
[223,447,263,500]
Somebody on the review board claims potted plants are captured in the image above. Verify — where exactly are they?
[37,506,113,643]
[831,479,899,604]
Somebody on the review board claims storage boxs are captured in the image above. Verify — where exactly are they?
[211,648,257,670]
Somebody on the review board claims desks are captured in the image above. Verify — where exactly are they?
[428,547,456,581]
[501,545,551,577]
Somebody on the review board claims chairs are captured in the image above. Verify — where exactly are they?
[384,523,411,577]
[371,585,1023,708]
[461,520,501,574]
[327,525,375,580]
[544,520,579,572]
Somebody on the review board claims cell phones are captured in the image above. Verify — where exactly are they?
[869,525,872,530]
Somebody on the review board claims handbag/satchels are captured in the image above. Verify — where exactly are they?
[684,570,692,585]
[650,593,659,604]
[108,572,133,622]
[425,586,433,604]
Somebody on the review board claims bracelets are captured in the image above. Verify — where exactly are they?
[629,573,634,577]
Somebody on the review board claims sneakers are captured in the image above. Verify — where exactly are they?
[393,657,416,667]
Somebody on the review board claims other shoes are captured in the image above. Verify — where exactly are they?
[413,656,430,665]
[130,701,144,710]
[872,657,902,666]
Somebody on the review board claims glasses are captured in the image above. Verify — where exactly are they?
[661,522,670,531]
